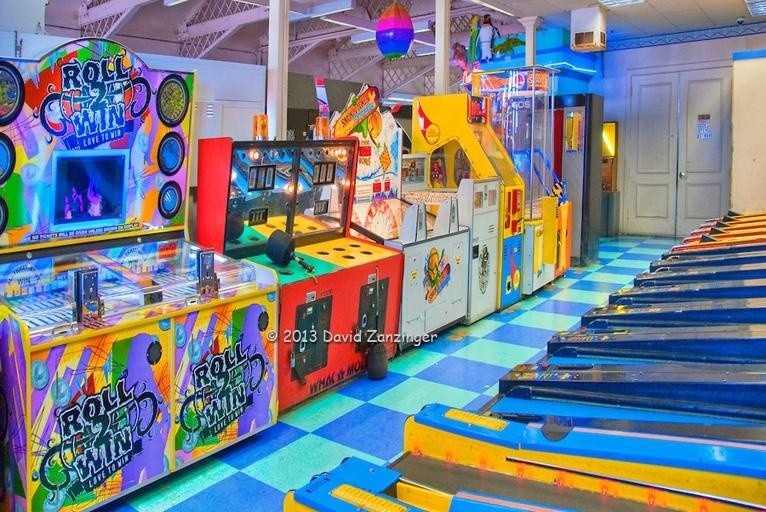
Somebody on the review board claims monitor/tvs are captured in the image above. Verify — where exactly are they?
[49,152,129,234]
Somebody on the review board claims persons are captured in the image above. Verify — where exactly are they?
[87,189,102,216]
[68,183,84,217]
[64,195,72,219]
